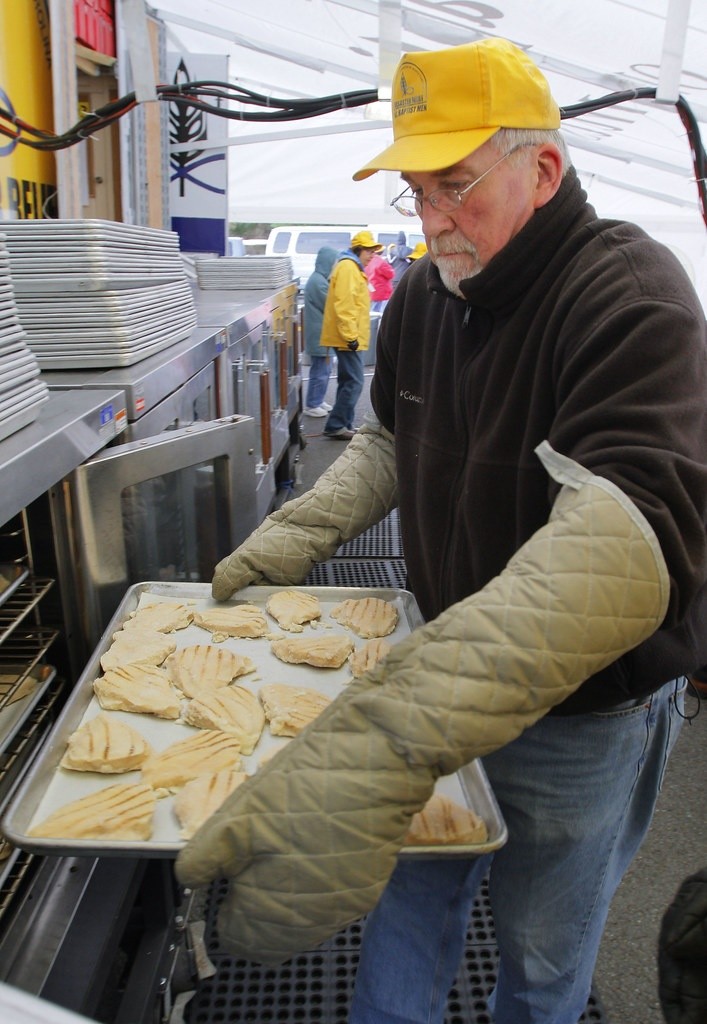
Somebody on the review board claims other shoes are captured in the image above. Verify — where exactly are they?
[321,426,359,440]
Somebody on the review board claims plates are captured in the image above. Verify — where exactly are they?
[0,223,50,443]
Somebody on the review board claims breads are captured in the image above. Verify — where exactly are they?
[22,590,490,845]
[0,674,39,708]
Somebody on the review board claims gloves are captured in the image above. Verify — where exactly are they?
[174,440,673,964]
[211,423,397,604]
[348,340,359,351]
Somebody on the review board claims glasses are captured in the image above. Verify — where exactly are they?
[390,142,544,217]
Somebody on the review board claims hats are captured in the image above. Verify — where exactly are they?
[406,243,427,259]
[352,37,561,181]
[349,229,382,251]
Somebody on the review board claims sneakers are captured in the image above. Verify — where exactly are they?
[303,406,328,417]
[317,402,333,411]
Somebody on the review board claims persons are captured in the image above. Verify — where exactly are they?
[174,43,707,1024]
[362,232,428,366]
[304,247,334,417]
[319,230,382,440]
